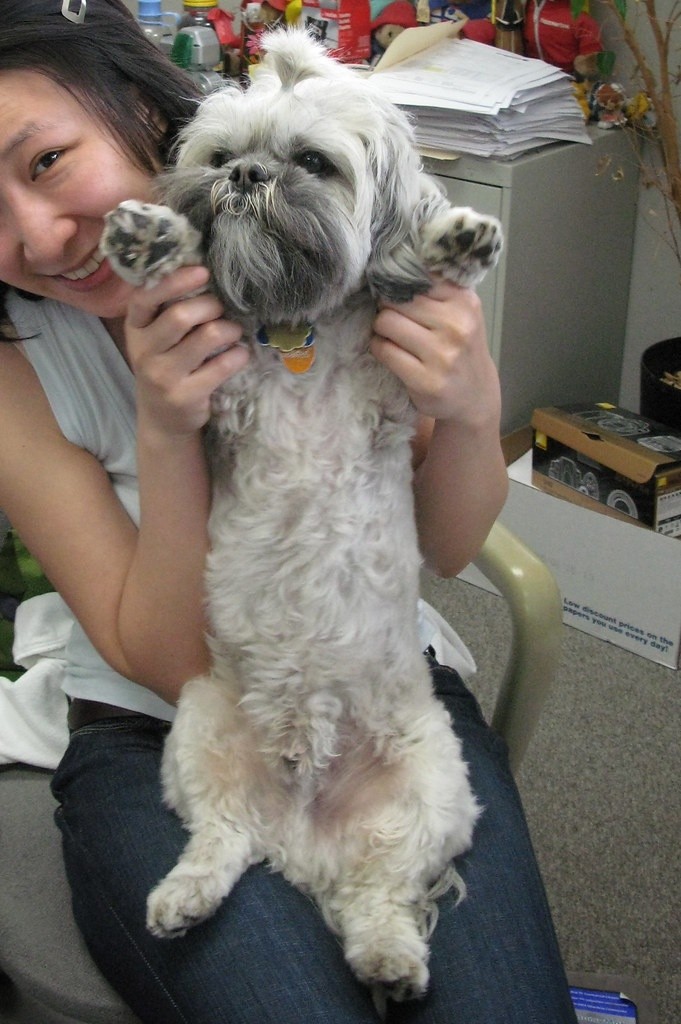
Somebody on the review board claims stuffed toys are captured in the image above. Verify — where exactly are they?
[370,0,657,129]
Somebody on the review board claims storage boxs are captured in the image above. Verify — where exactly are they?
[452,448,681,670]
[527,397,681,539]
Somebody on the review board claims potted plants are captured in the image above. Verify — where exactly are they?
[569,0,681,438]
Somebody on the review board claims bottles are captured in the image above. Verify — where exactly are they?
[241,3,264,88]
[137,0,180,56]
[177,0,225,77]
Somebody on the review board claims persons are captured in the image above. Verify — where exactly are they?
[0,0,580,1023]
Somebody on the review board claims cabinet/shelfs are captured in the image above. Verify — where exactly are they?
[412,120,645,439]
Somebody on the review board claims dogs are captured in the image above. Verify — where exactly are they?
[101,27,503,1008]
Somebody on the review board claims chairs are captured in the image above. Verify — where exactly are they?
[0,521,565,1023]
